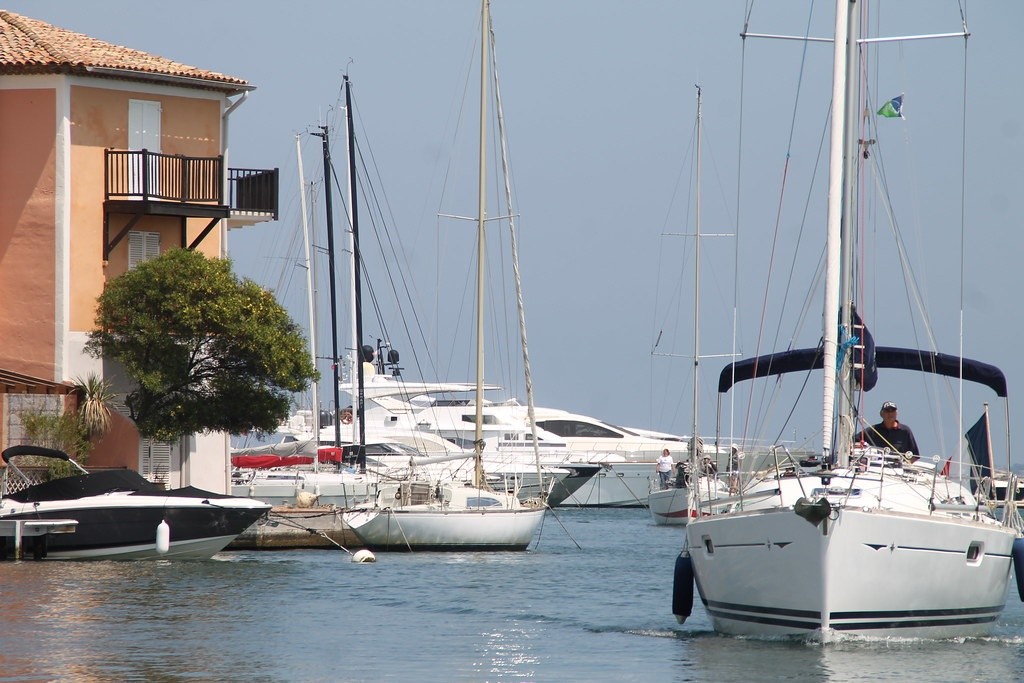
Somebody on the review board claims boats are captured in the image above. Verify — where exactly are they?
[0,442,272,567]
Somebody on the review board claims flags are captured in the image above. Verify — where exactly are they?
[965,410,995,501]
[877,92,906,120]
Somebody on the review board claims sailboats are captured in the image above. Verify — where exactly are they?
[674,1,1017,640]
[221,4,717,523]
[340,3,554,555]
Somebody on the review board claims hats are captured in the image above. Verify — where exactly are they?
[703,455,711,460]
[881,401,897,410]
[732,444,738,451]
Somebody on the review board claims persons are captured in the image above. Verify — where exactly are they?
[854,401,920,463]
